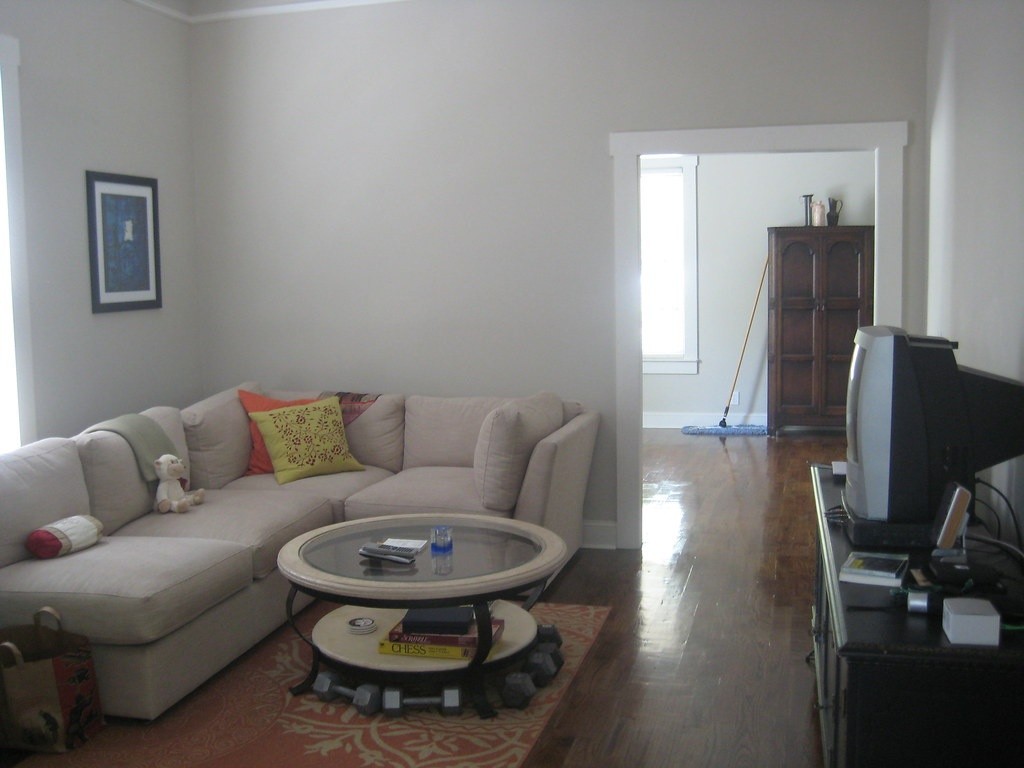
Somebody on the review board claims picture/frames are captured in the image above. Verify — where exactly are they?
[85,169,164,315]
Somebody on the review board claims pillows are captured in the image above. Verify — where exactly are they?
[235,391,318,477]
[0,380,580,571]
[27,515,104,559]
[249,397,366,486]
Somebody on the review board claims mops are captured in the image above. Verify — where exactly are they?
[680,257,767,436]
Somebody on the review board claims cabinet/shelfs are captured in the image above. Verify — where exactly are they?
[766,228,872,441]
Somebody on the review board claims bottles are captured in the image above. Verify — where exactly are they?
[811,200,825,226]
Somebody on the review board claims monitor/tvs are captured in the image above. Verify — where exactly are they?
[843,326,1024,521]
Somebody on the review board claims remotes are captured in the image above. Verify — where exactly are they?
[359,543,418,565]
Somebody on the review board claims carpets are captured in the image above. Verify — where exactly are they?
[13,601,611,768]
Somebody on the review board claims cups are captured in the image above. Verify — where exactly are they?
[429,524,453,556]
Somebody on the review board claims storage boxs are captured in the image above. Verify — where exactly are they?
[943,599,1001,646]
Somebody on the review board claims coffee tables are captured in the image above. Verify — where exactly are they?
[277,511,571,717]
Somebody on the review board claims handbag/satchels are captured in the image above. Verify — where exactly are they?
[0,607,108,756]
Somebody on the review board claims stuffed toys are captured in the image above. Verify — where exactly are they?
[153,454,205,513]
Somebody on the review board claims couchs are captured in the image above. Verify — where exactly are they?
[0,383,603,725]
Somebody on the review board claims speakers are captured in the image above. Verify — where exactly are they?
[930,482,971,557]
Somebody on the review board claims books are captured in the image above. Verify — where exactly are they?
[839,553,910,587]
[378,606,505,660]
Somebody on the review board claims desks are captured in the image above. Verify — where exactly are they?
[809,461,1024,768]
